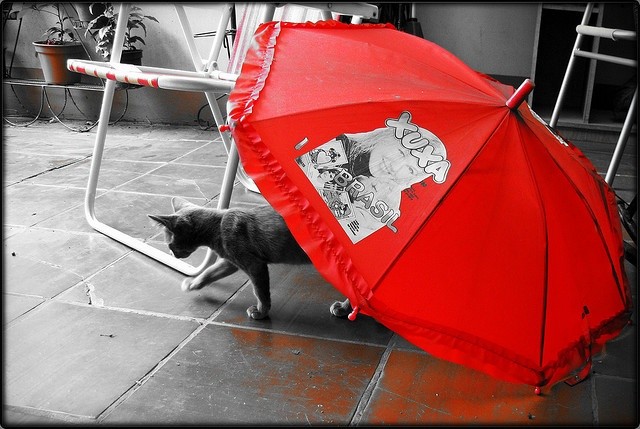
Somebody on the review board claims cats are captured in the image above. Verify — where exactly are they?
[147,195,353,320]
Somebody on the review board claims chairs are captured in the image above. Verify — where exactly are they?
[84,3,380,277]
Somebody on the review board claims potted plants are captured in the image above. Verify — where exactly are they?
[16,2,86,84]
[73,2,160,90]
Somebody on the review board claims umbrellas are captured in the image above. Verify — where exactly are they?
[218,22,633,393]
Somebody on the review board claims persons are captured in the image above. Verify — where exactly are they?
[344,123,448,189]
[303,165,340,189]
